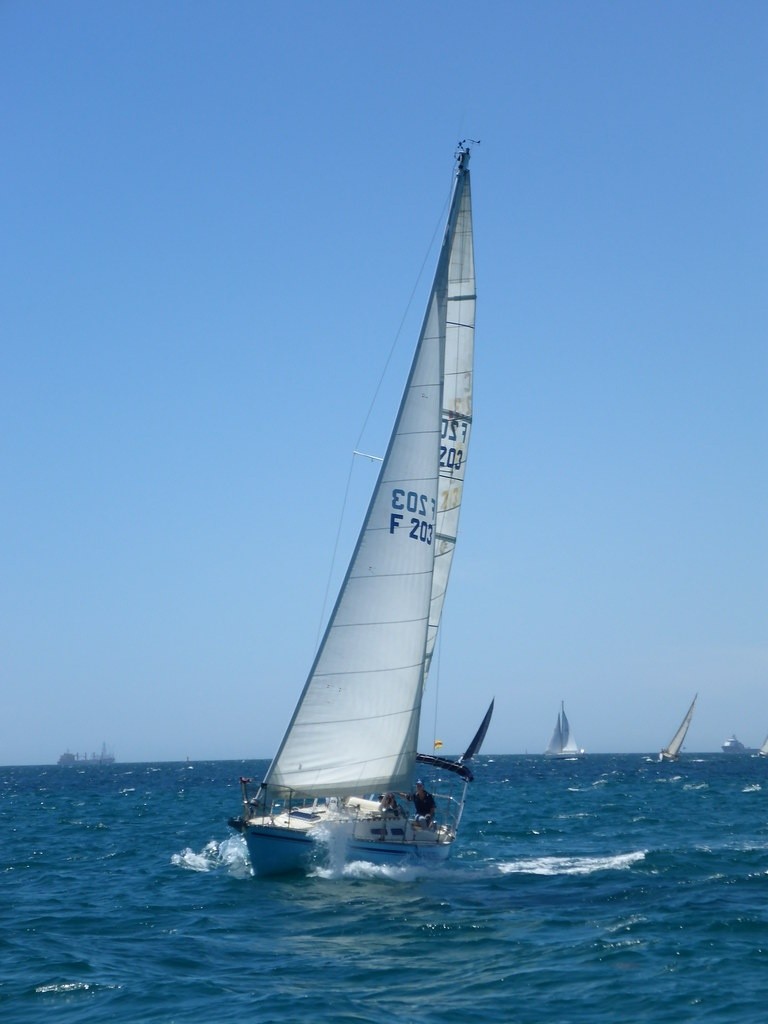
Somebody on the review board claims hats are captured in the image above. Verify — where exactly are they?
[416,778,425,785]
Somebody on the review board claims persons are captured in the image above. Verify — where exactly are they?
[399,777,438,834]
[377,792,408,817]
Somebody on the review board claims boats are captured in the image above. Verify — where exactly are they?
[57,742,116,767]
[720,734,762,756]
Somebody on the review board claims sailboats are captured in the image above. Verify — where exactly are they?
[658,694,699,763]
[232,146,485,882]
[547,700,584,766]
[465,698,495,761]
